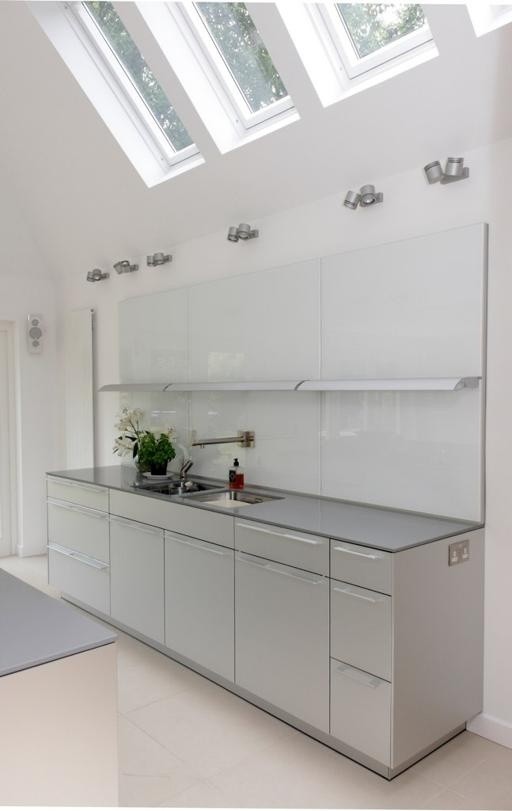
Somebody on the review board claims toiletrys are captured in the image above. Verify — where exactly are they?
[228,457,244,490]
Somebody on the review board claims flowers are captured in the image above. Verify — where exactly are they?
[112,403,154,459]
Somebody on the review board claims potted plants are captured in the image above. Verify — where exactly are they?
[138,429,177,474]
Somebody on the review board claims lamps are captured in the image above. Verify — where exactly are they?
[226,221,262,245]
[341,151,473,210]
[84,250,172,284]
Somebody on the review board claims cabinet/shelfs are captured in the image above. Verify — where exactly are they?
[233,509,329,743]
[45,471,109,623]
[108,483,235,695]
[330,522,484,781]
[0,568,119,806]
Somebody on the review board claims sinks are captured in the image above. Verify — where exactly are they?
[126,477,228,496]
[177,486,286,508]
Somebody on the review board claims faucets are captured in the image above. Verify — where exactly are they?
[180,459,193,478]
